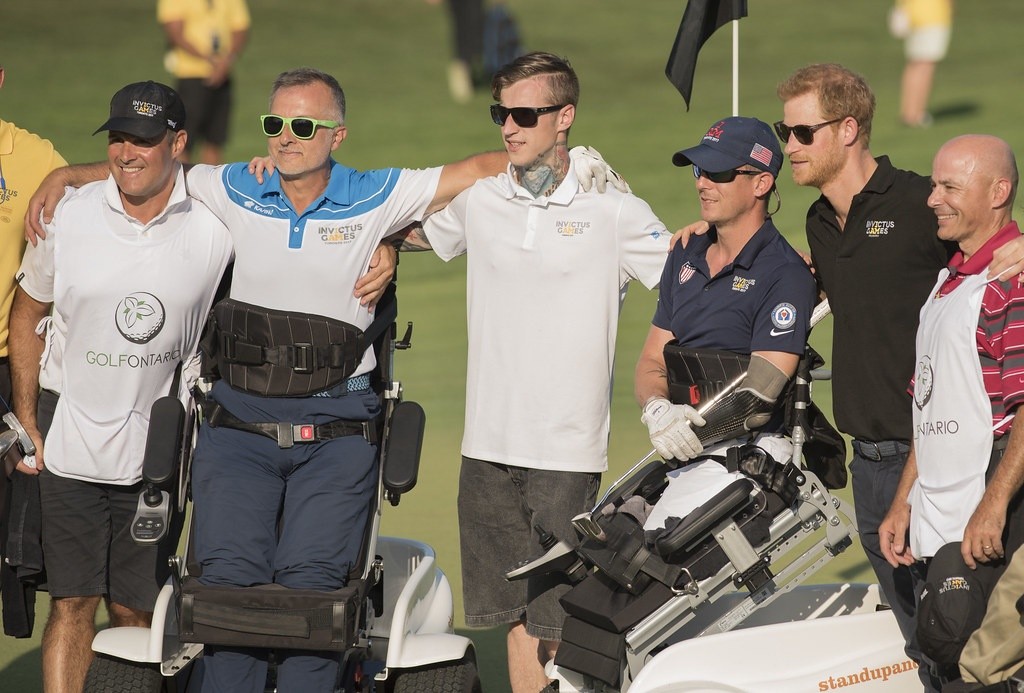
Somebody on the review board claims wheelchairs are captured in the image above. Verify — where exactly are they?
[84,274,480,693]
[504,338,920,693]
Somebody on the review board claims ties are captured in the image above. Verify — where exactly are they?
[773,120,839,145]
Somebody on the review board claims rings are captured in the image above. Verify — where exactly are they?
[981,544,993,549]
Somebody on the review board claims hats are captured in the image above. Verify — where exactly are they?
[918,541,988,664]
[672,116,784,178]
[92,80,186,145]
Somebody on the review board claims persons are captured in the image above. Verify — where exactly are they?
[0,68,71,422]
[5,81,397,693]
[632,115,816,462]
[21,68,631,693]
[879,132,1024,571]
[247,52,816,693]
[435,0,487,105]
[667,67,1024,693]
[156,0,252,167]
[888,1,956,130]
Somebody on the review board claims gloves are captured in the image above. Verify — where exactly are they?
[641,398,706,462]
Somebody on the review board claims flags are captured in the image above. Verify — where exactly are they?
[664,0,748,115]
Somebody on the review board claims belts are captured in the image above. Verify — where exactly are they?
[315,376,370,397]
[853,439,910,462]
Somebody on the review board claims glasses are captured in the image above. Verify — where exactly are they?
[490,103,565,127]
[692,164,763,183]
[260,116,339,140]
